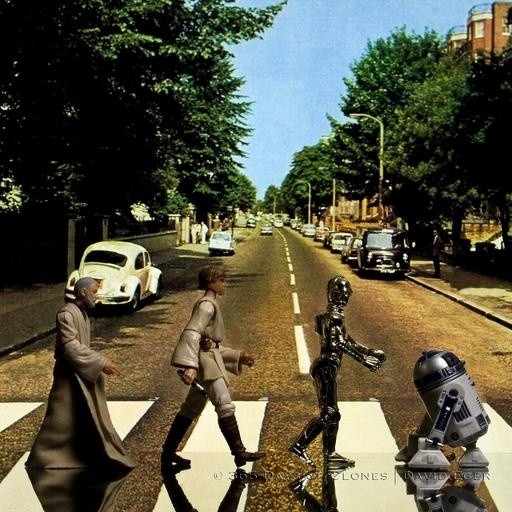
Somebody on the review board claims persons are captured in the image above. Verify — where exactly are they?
[161,266,266,469]
[200,221,208,243]
[24,277,137,475]
[288,274,389,464]
[430,228,445,277]
